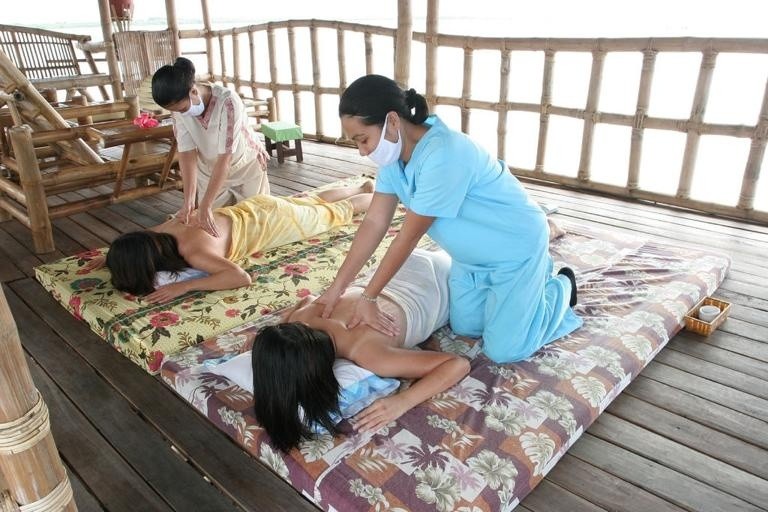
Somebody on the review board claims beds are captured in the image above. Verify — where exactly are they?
[159,216,730,512]
[32,174,447,376]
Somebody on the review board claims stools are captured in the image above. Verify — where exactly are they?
[261,121,305,164]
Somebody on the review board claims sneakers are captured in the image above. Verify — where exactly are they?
[558,267,577,306]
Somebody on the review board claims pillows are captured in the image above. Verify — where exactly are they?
[203,350,401,436]
[153,267,209,288]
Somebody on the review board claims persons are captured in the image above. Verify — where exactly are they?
[151,57,271,238]
[249,217,568,456]
[310,74,584,365]
[84,179,375,305]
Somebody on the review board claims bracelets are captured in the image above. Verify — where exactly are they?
[359,291,379,303]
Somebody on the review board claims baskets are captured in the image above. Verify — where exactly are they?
[683,295,732,337]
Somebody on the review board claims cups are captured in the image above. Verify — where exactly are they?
[698,306,720,323]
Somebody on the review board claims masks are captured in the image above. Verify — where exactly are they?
[367,112,403,168]
[180,87,205,117]
